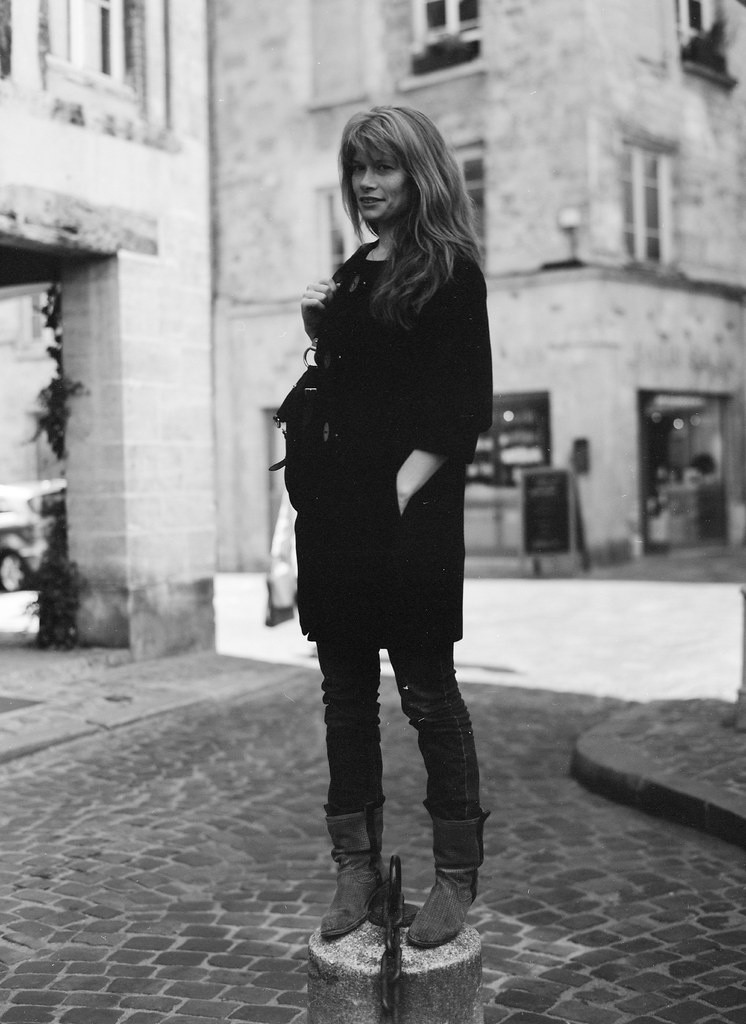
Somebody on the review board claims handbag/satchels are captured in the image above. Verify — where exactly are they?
[268,242,369,513]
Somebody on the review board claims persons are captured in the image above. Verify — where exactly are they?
[294,105,493,950]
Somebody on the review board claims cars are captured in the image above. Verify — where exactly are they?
[0,479,68,593]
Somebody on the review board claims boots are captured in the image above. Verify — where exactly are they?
[408,799,491,948]
[320,797,389,937]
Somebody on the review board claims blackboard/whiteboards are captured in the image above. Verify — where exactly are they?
[521,468,578,555]
